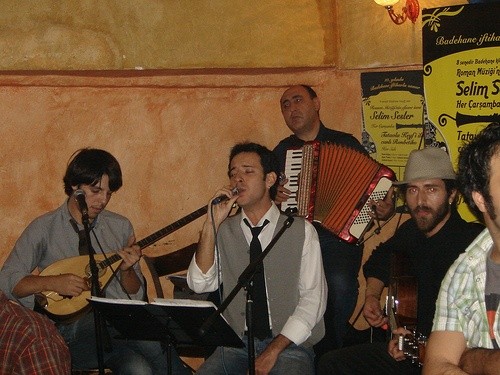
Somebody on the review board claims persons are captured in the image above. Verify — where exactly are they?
[318,149,488,375]
[0,290,72,374]
[271,84,397,347]
[421,120,500,375]
[0,148,187,375]
[187,142,328,375]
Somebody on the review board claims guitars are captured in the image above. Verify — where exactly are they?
[385,265,428,369]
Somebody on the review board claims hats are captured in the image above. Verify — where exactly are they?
[392,147,457,184]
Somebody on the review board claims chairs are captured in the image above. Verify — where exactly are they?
[143,242,207,358]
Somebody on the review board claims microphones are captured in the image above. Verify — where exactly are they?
[212,188,239,204]
[74,189,88,215]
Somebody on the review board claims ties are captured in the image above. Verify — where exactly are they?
[69,218,97,255]
[243,218,271,342]
